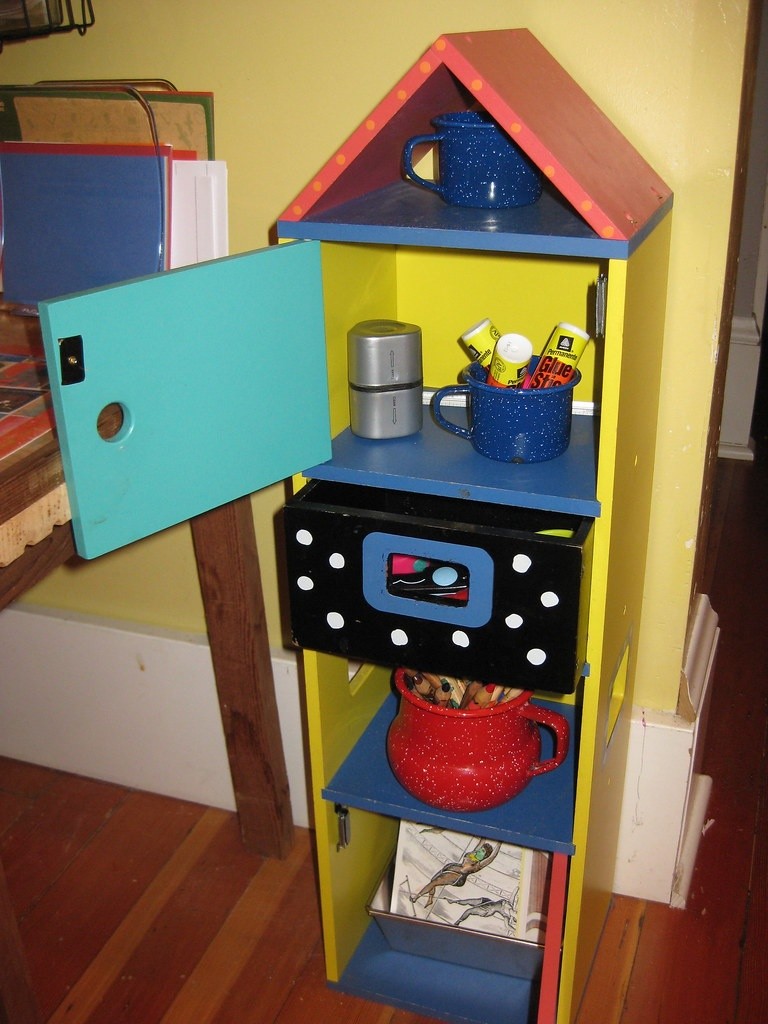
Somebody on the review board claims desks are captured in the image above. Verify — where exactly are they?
[0,292,297,862]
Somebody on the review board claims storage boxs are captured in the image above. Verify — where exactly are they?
[366,843,545,981]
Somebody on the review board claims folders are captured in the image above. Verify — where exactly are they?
[0,152,169,308]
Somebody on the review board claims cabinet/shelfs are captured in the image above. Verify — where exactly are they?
[38,28,674,1024]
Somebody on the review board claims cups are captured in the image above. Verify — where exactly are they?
[432,356,582,463]
[403,112,542,208]
[386,667,570,814]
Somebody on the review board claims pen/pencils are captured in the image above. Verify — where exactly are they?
[399,669,525,712]
